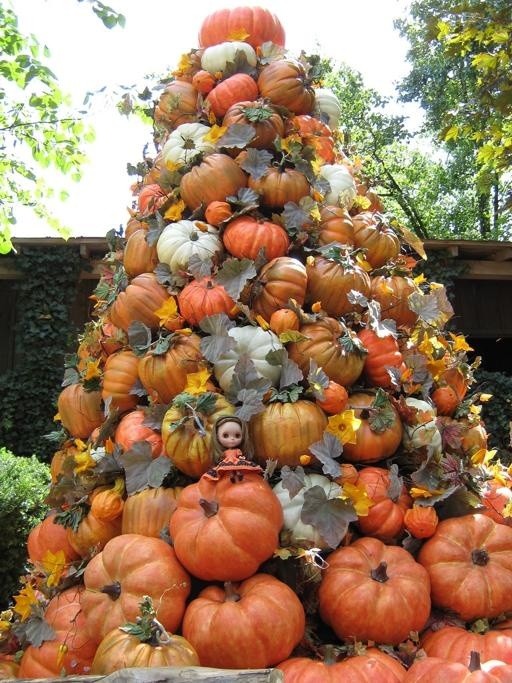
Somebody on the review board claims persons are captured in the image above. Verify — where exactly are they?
[202,413,266,482]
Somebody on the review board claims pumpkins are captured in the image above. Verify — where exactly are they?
[2,8,511,682]
